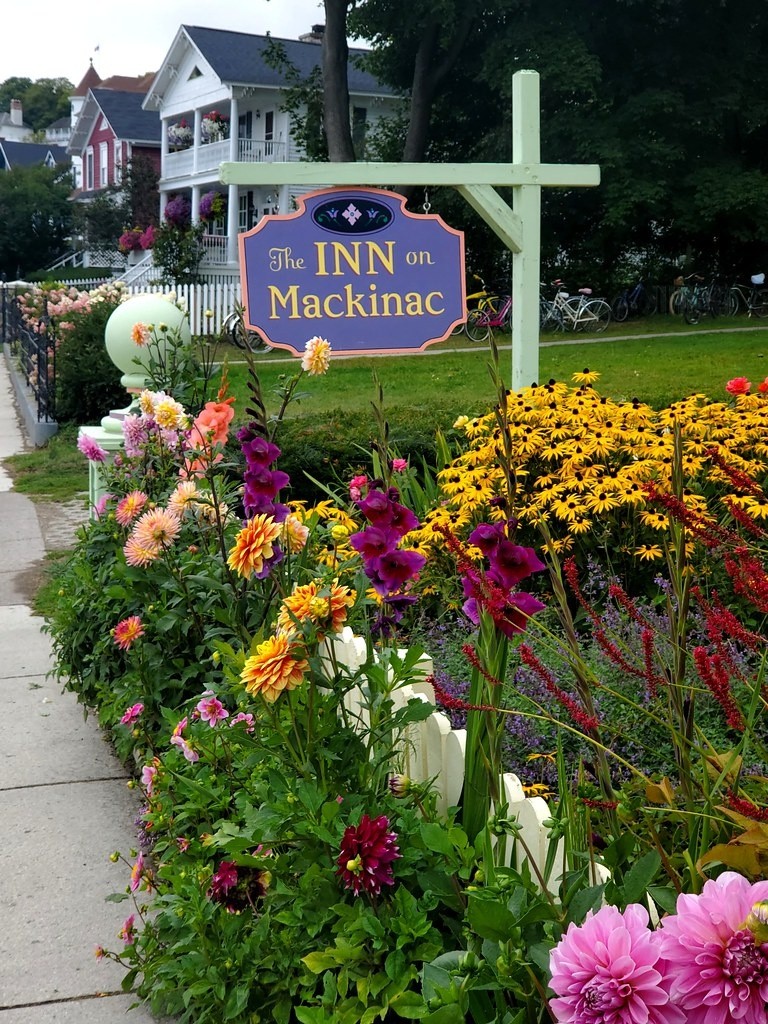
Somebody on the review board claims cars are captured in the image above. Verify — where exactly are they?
[63,231,88,241]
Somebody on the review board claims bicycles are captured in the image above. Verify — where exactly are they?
[223,313,276,354]
[669,271,768,325]
[450,275,513,343]
[611,276,658,322]
[539,279,612,334]
